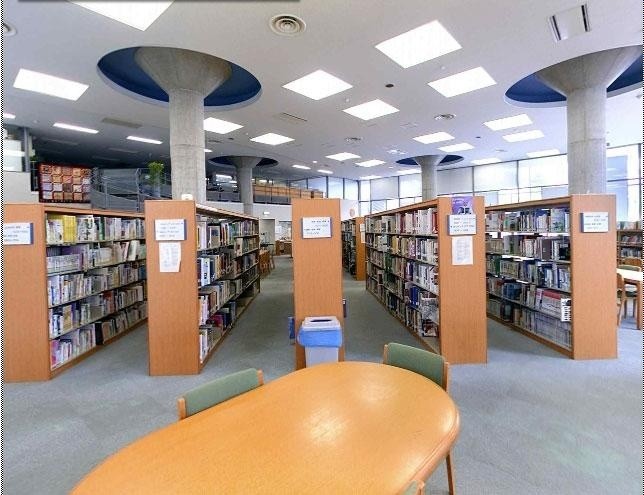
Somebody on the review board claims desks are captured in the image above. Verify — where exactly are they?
[63,360,463,495]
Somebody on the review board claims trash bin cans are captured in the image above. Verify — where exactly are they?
[297,316,342,368]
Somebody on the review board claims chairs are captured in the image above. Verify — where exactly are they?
[615,255,644,332]
[258,247,275,276]
[401,479,427,495]
[382,342,456,495]
[173,365,267,421]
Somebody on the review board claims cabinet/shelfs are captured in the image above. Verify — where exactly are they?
[363,194,489,366]
[289,196,347,371]
[341,216,368,282]
[1,202,147,382]
[484,193,617,360]
[144,200,262,376]
[616,228,644,259]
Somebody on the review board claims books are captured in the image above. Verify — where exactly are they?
[195,213,258,364]
[341,208,440,337]
[46,214,147,370]
[615,220,643,258]
[484,207,570,349]
